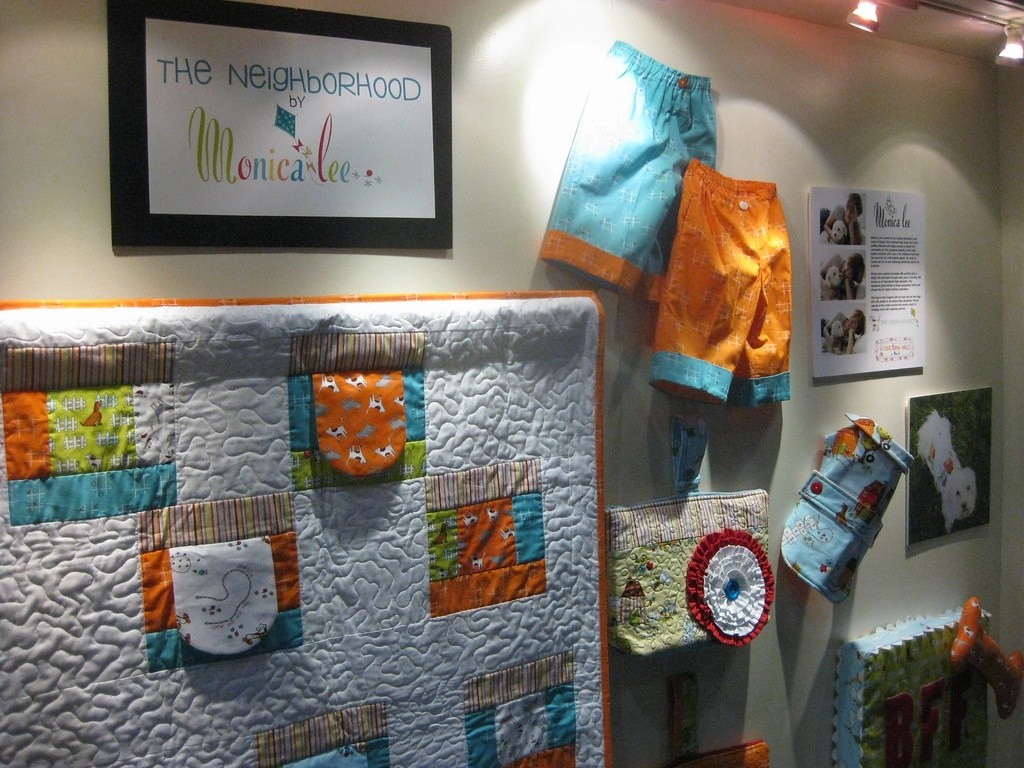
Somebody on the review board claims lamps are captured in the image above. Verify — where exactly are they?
[848,0,881,33]
[998,24,1024,58]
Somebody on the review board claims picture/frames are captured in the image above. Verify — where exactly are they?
[106,0,453,253]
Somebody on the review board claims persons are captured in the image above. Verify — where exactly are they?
[821,309,866,354]
[824,192,862,245]
[820,252,864,300]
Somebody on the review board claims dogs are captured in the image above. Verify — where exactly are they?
[821,320,847,356]
[821,265,842,301]
[918,409,979,536]
[819,219,848,246]
